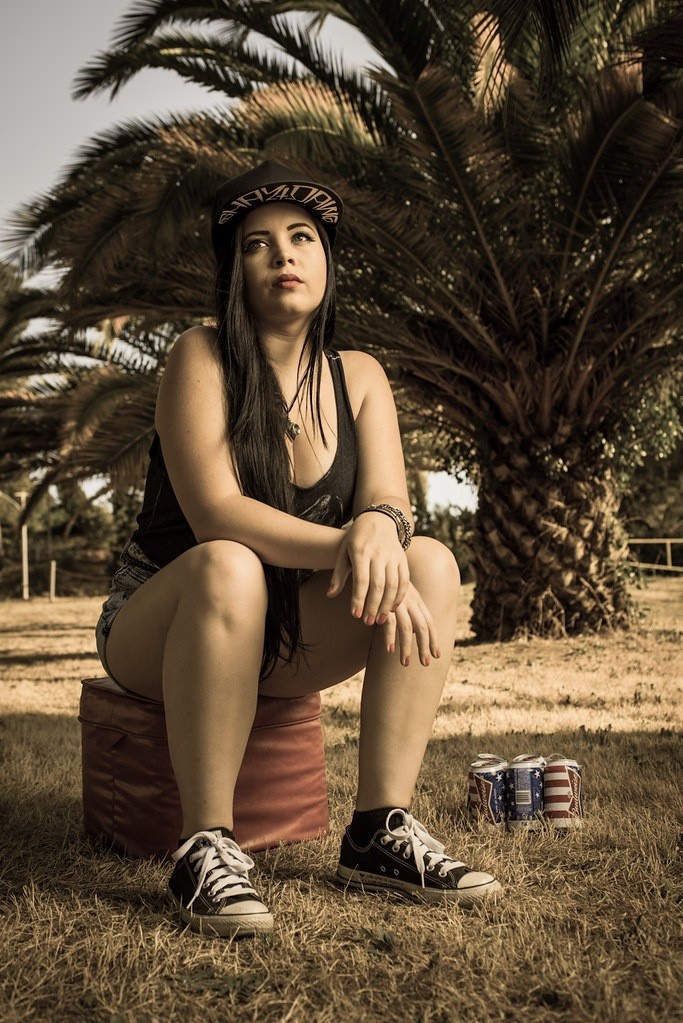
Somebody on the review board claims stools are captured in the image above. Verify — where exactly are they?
[76,677,330,862]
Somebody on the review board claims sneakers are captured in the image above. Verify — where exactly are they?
[335,809,506,907]
[165,826,273,935]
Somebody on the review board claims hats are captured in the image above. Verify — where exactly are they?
[209,160,344,243]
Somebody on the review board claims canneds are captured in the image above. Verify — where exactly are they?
[466,752,583,831]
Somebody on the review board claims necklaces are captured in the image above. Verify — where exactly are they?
[280,366,310,443]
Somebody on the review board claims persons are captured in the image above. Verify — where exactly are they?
[96,160,504,938]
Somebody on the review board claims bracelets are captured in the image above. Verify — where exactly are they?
[353,504,412,552]
